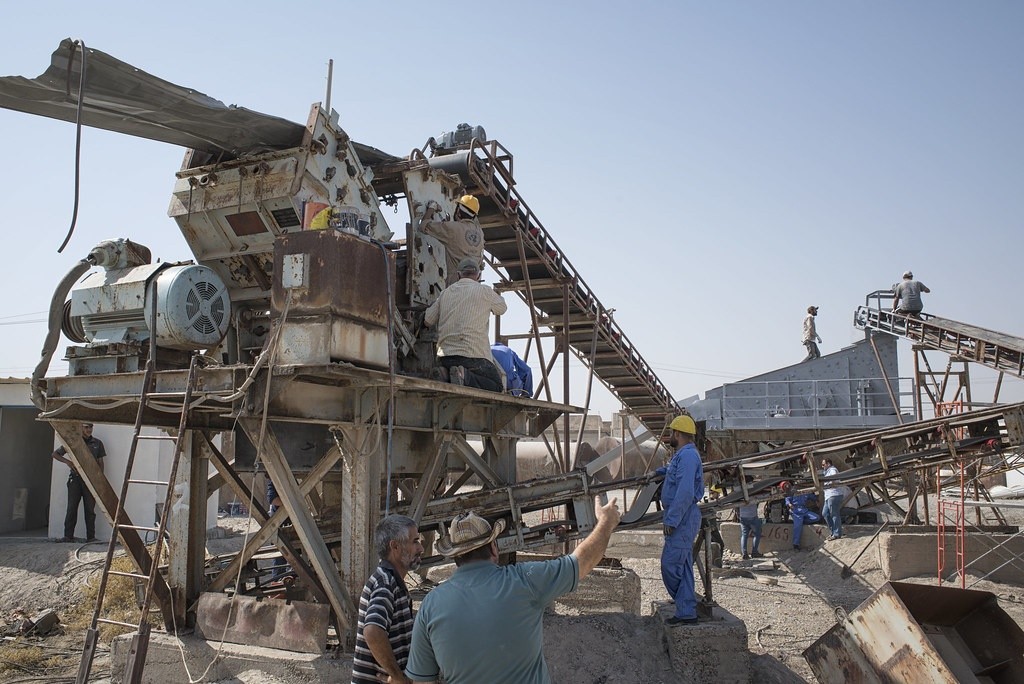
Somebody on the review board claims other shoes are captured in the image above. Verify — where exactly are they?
[742,555,749,560]
[749,552,764,558]
[88,538,101,544]
[54,537,74,543]
[825,535,840,541]
[793,544,800,552]
[447,366,462,386]
[430,365,447,382]
[665,615,698,627]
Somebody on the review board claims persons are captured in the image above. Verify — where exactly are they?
[424,258,507,393]
[267,479,300,585]
[892,271,930,328]
[52,423,107,544]
[351,514,423,684]
[405,496,620,684]
[764,480,788,522]
[785,475,820,552]
[705,485,724,558]
[489,342,533,398]
[840,486,859,524]
[642,415,704,627]
[418,195,484,287]
[802,306,822,361]
[740,475,763,559]
[821,458,842,541]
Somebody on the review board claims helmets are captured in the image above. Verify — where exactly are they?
[667,414,695,435]
[457,195,480,215]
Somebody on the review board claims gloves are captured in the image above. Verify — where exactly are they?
[427,200,442,213]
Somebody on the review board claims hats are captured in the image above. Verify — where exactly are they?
[807,307,819,313]
[434,510,507,557]
[458,258,480,274]
[903,271,913,278]
